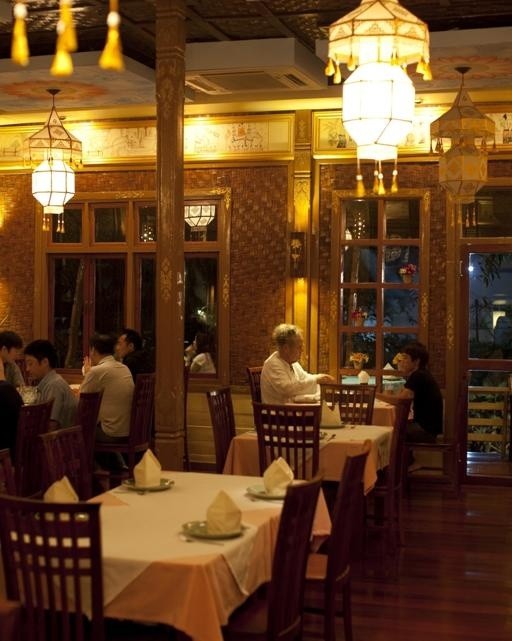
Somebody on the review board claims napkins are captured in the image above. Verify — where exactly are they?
[44,475,78,502]
[358,371,370,382]
[318,400,341,425]
[203,492,243,532]
[132,449,163,484]
[261,457,293,494]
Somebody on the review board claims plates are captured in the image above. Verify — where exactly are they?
[246,482,289,499]
[122,477,175,491]
[182,520,245,540]
[318,422,345,429]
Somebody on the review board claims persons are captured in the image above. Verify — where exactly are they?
[375,343,443,490]
[80,334,135,443]
[185,332,217,375]
[260,323,335,405]
[23,339,79,433]
[84,329,154,380]
[0,330,25,386]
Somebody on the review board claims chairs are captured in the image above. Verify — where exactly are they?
[247,366,262,403]
[40,425,93,500]
[320,381,377,426]
[374,397,411,556]
[17,397,57,459]
[98,373,156,485]
[229,475,323,641]
[1,448,20,494]
[307,448,370,640]
[400,390,451,498]
[205,387,235,474]
[75,387,106,434]
[1,494,106,639]
[251,398,322,478]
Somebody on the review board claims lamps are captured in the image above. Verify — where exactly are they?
[185,205,217,240]
[325,0,431,204]
[26,88,82,233]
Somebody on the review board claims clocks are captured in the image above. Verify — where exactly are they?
[376,232,406,265]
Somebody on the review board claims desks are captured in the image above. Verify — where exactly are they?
[8,468,334,640]
[285,390,400,429]
[340,373,404,391]
[20,383,87,446]
[227,423,395,496]
